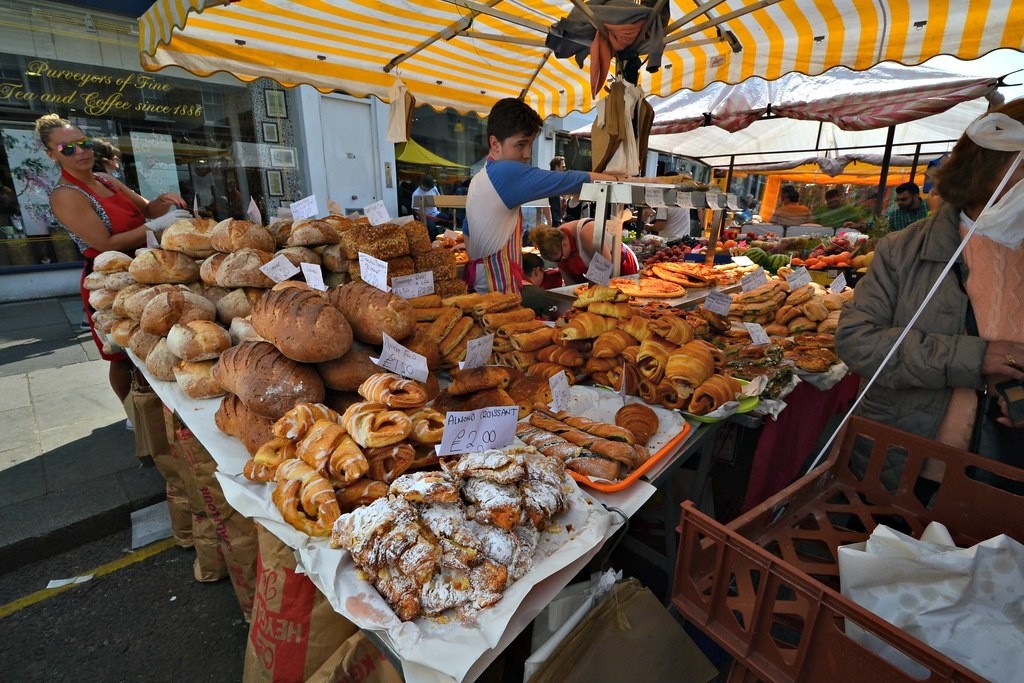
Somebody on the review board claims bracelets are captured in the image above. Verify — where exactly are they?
[613,175,619,182]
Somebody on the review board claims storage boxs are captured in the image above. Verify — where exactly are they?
[684,253,732,265]
[785,225,834,237]
[742,225,784,237]
[668,415,1024,683]
[791,264,854,286]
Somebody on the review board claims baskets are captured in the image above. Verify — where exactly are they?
[671,414,1024,683]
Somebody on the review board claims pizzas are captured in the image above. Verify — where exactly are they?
[575,260,742,296]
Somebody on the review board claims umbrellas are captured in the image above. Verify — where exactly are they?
[134,0,1023,211]
[566,49,1024,271]
[396,137,492,187]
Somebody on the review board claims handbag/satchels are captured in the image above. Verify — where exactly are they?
[967,379,1024,495]
[923,152,950,194]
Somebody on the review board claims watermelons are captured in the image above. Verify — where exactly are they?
[739,247,790,270]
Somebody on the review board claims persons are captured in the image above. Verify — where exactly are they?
[886,182,930,232]
[529,217,639,287]
[397,174,473,226]
[522,253,556,322]
[80,138,120,331]
[412,155,811,241]
[180,179,245,221]
[812,99,1024,535]
[463,97,665,296]
[856,193,878,227]
[812,190,865,229]
[35,113,192,432]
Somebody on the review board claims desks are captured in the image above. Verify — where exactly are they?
[543,270,742,311]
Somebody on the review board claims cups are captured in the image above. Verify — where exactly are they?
[0,226,14,235]
[10,216,23,230]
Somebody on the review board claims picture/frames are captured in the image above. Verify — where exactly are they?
[265,169,285,197]
[269,147,297,168]
[261,121,280,144]
[279,199,295,208]
[263,88,289,119]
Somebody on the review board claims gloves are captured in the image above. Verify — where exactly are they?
[144,210,192,233]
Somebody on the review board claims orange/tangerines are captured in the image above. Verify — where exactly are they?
[791,251,850,268]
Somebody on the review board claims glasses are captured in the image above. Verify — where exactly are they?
[894,195,914,201]
[562,166,565,168]
[49,137,93,156]
[829,199,839,205]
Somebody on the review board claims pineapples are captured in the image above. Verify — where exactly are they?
[864,215,889,253]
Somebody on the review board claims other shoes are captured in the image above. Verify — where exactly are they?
[80,320,92,328]
[126,420,133,429]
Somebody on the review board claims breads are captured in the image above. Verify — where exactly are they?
[550,287,743,415]
[83,223,657,623]
[642,301,843,373]
[709,263,859,335]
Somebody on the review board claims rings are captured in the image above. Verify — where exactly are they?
[1004,352,1015,366]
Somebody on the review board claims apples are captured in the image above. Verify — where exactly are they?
[645,243,691,264]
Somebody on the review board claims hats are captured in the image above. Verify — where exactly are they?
[421,176,436,188]
